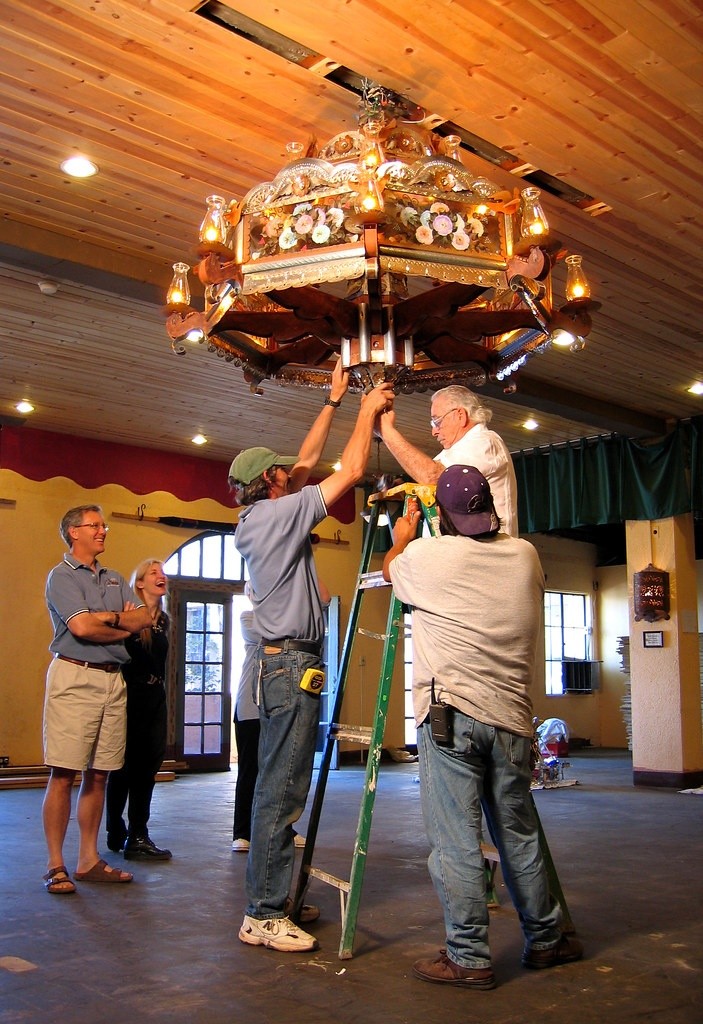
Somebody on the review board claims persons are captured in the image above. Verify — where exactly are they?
[106,559,172,860]
[373,385,518,538]
[382,464,584,990]
[227,357,395,951]
[42,504,152,892]
[232,580,307,850]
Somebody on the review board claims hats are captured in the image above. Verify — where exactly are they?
[436,464,498,536]
[228,447,301,491]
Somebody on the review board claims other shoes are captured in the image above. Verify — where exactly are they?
[231,837,248,850]
[293,833,308,848]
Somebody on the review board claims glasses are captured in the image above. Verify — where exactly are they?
[430,407,462,428]
[73,522,111,533]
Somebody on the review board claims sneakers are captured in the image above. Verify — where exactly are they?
[123,837,172,861]
[284,898,319,925]
[238,914,319,952]
[410,947,495,990]
[105,828,124,852]
[522,940,583,970]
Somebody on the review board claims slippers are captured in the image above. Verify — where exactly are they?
[73,860,132,883]
[43,864,77,892]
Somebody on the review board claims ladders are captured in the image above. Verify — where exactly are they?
[292,484,502,958]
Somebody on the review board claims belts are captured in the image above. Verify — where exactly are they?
[55,651,119,673]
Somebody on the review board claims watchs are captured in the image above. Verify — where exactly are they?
[324,395,341,408]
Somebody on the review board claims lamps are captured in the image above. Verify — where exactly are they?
[634,564,670,623]
[164,78,602,527]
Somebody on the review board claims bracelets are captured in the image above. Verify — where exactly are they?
[111,611,120,627]
